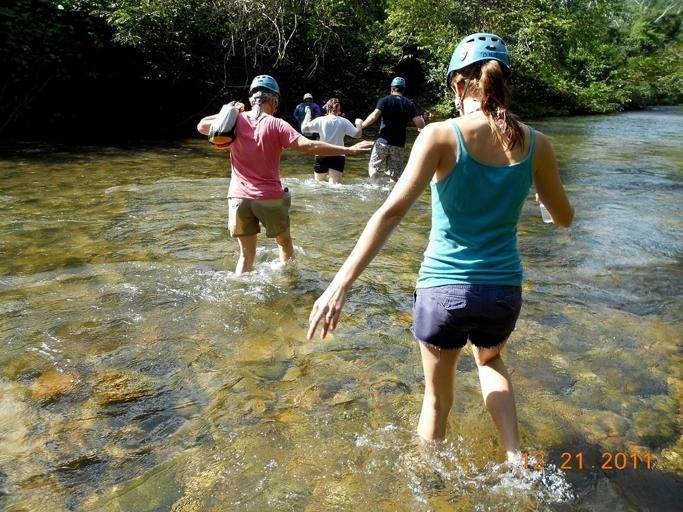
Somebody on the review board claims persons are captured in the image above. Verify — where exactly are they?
[293,93,321,140]
[195,74,377,274]
[361,77,425,183]
[300,97,364,185]
[305,31,574,476]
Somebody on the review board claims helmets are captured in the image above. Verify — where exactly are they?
[249,75,280,96]
[302,93,312,100]
[390,76,406,89]
[445,32,511,92]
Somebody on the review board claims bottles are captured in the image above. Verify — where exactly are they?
[539,203,553,222]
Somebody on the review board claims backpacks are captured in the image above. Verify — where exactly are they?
[298,102,314,127]
